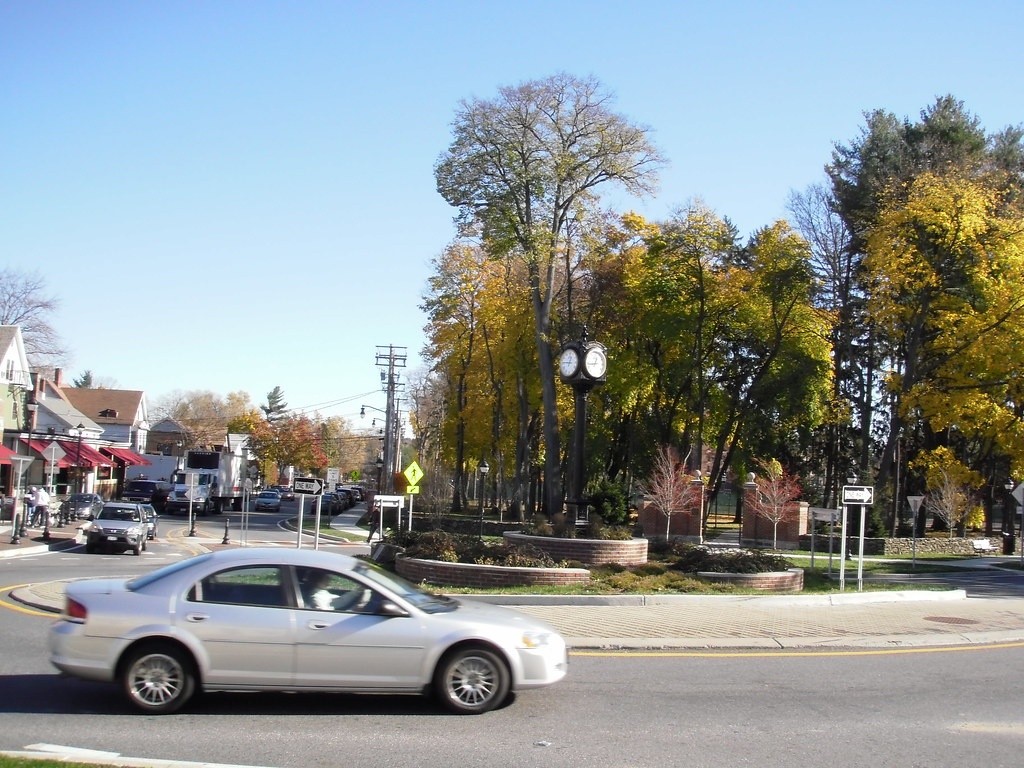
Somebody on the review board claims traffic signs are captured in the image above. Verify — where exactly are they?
[293,477,324,496]
[842,486,873,504]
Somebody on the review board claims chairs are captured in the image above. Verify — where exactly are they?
[283,570,310,608]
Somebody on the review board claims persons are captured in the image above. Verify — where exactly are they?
[304,568,341,611]
[364,507,380,543]
[25,487,49,528]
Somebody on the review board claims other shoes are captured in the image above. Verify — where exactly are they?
[40,526,46,529]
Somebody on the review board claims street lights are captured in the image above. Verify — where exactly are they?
[19,392,39,538]
[360,404,386,419]
[73,420,86,521]
[372,418,386,428]
[478,450,489,542]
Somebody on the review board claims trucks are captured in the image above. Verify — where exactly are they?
[165,450,246,516]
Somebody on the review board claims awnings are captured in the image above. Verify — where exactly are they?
[0,443,19,465]
[20,438,117,468]
[102,447,152,465]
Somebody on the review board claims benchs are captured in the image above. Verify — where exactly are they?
[971,540,999,557]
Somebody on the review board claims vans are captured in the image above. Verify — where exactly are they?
[121,480,171,514]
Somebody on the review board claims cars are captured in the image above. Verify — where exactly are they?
[48,547,568,715]
[311,486,366,515]
[140,504,157,540]
[58,493,104,520]
[87,503,147,555]
[279,488,294,501]
[255,491,281,512]
